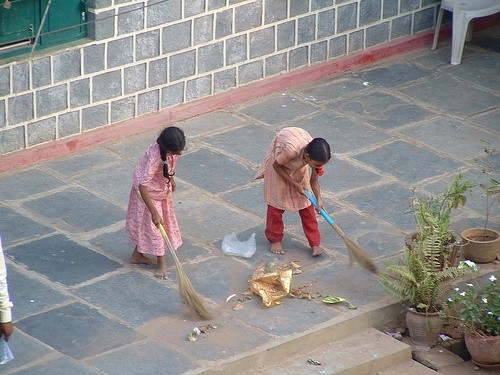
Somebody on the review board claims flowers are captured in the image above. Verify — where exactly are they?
[438,260,500,334]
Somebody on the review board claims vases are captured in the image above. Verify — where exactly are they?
[464,330,500,368]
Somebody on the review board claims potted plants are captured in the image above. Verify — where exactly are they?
[377,146,500,346]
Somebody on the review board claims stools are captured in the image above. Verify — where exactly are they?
[431,0,500,65]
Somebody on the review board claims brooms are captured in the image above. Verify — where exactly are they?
[137,188,221,319]
[303,189,377,274]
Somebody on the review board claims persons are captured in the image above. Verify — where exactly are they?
[0,238,15,366]
[126,127,186,279]
[252,127,331,256]
